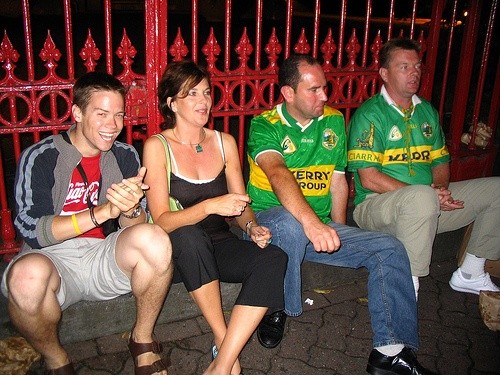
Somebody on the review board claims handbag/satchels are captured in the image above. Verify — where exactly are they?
[148,134,185,236]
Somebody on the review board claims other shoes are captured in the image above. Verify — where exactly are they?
[212,344,244,375]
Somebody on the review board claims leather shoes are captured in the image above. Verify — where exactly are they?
[256,311,286,348]
[367,345,437,375]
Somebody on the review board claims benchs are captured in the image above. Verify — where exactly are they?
[56,208,467,347]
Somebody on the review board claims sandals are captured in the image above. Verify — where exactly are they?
[127,322,172,375]
[42,356,78,375]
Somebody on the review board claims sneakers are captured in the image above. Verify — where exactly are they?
[450,268,500,296]
[414,281,419,300]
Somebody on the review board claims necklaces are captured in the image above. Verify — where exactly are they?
[172,126,206,153]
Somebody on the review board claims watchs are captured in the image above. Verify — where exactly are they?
[244,218,257,236]
[119,204,143,219]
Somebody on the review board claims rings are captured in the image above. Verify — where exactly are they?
[266,237,274,244]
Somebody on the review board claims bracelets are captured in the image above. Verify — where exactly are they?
[89,206,102,228]
[71,214,83,235]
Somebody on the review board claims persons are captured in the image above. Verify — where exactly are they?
[348,39,500,308]
[1,69,175,374]
[142,57,289,375]
[239,54,434,375]
